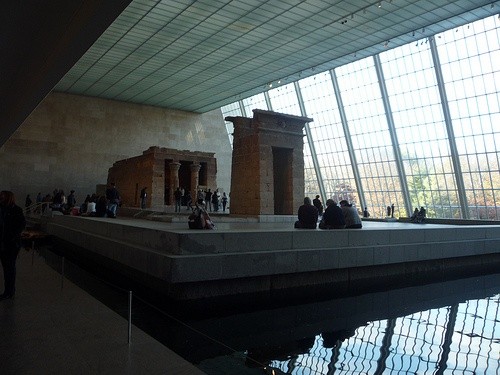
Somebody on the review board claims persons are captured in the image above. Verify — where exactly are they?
[339,200,362,229]
[313,195,323,216]
[84,183,122,220]
[410,207,426,224]
[174,188,183,212]
[196,187,228,212]
[364,208,368,217]
[0,190,26,304]
[24,187,79,217]
[294,196,319,230]
[186,190,194,211]
[319,199,345,229]
[387,203,394,218]
[140,186,148,210]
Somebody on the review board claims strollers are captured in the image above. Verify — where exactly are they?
[188,201,214,230]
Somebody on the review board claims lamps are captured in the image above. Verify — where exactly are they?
[378,1,381,8]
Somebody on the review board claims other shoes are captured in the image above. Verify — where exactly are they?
[0,294,14,300]
